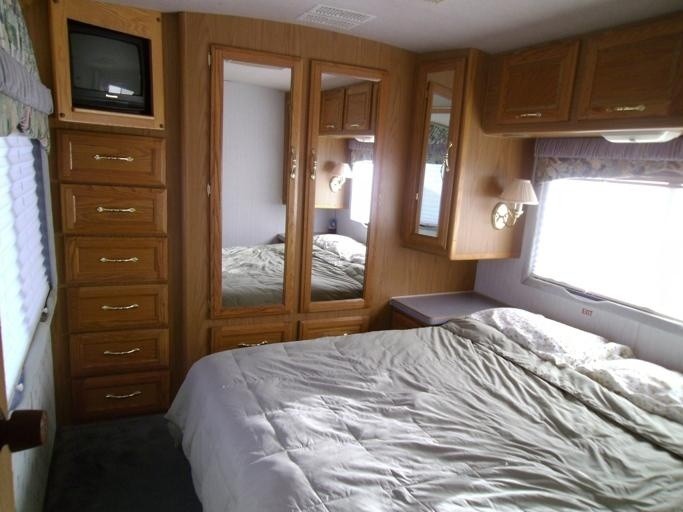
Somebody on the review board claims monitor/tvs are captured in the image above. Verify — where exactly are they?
[67,17,154,117]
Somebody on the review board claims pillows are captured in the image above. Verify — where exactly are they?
[312,232,367,266]
[475,309,682,424]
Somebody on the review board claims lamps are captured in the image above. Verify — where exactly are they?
[330,163,354,193]
[492,177,538,233]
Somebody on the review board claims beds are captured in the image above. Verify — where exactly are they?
[182,308,682,512]
[220,238,365,307]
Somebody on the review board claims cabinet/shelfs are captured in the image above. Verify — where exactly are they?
[401,49,535,261]
[185,13,397,320]
[52,125,174,421]
[320,84,371,133]
[485,15,683,132]
[208,316,372,352]
[284,91,349,209]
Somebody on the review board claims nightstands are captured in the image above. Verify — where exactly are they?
[389,292,509,330]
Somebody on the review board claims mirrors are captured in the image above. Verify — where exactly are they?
[405,57,467,247]
[310,62,386,309]
[218,49,293,314]
